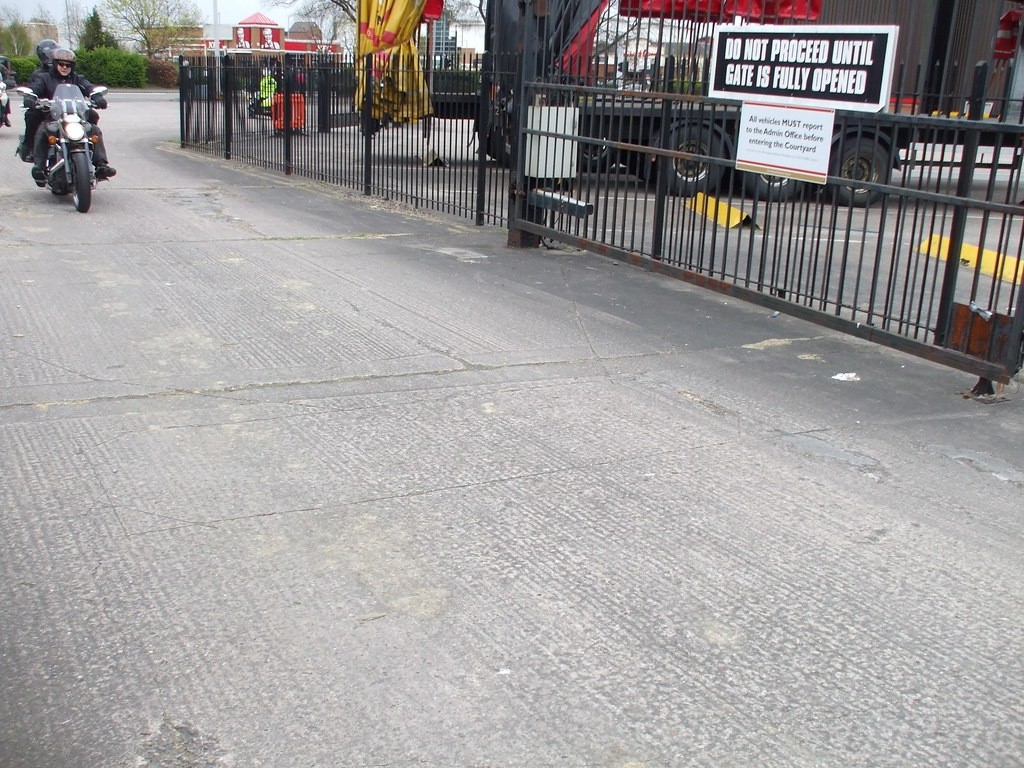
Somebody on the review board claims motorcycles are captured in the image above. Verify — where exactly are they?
[16,86,108,214]
[0,56,17,128]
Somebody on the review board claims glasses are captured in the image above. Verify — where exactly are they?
[58,60,72,68]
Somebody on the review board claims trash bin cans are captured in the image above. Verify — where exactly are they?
[269,91,310,137]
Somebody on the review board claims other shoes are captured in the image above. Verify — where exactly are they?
[94,166,116,178]
[4,119,11,127]
[31,165,47,187]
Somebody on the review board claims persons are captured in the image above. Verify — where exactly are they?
[23,39,116,188]
[260,68,276,107]
[0,55,14,127]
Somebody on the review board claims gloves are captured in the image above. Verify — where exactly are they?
[23,94,37,111]
[90,93,108,110]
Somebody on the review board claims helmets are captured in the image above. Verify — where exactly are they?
[52,48,77,67]
[34,38,59,64]
